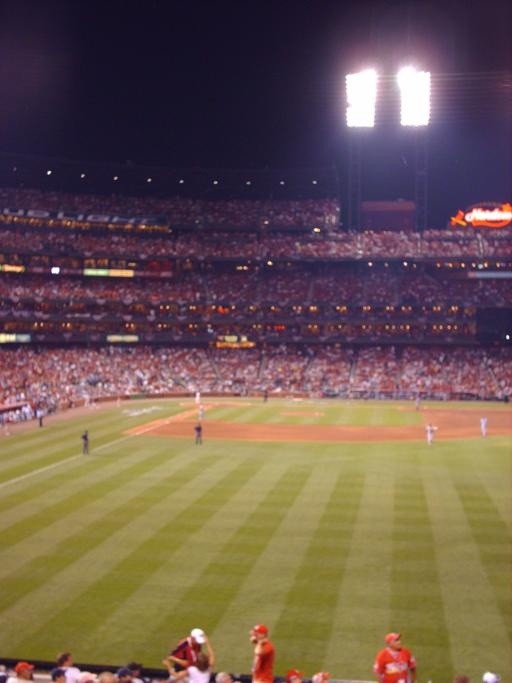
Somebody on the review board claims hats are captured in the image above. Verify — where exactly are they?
[118,662,142,675]
[51,669,68,677]
[15,661,35,673]
[191,629,206,644]
[384,632,401,644]
[254,624,268,633]
[285,669,330,683]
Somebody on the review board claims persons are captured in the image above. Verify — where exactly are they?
[372,633,416,683]
[194,423,203,446]
[81,430,89,455]
[453,673,470,683]
[162,629,215,683]
[482,671,501,683]
[36,409,46,428]
[480,416,488,436]
[425,424,439,446]
[249,623,276,683]
